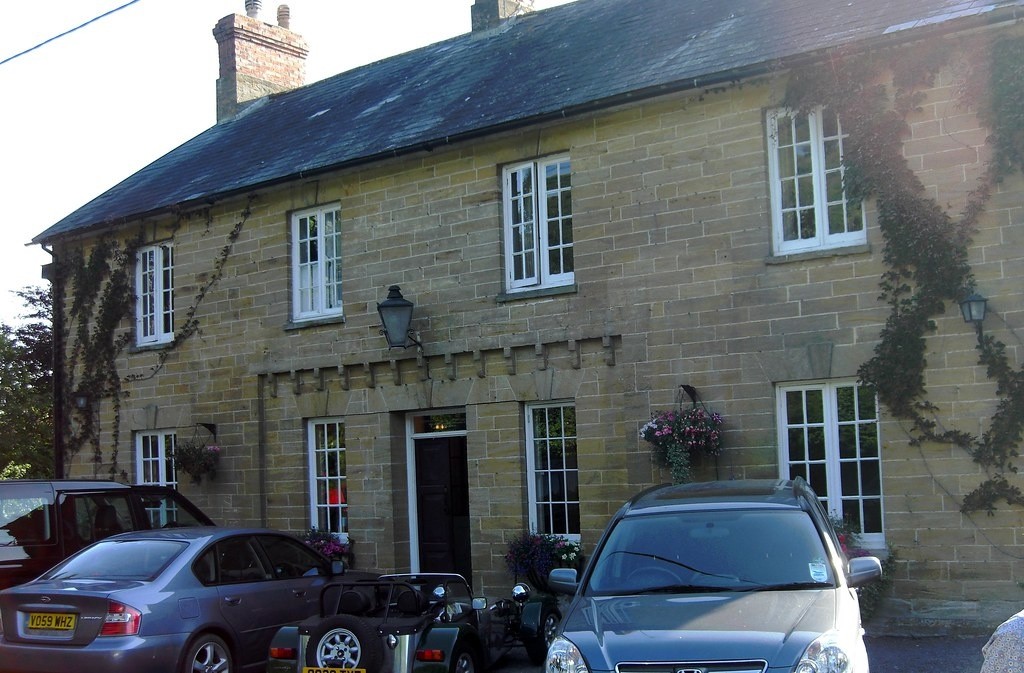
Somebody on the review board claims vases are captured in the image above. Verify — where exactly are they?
[525,561,581,591]
[655,444,693,467]
[184,460,207,474]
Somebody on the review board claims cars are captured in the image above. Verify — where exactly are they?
[0,525,339,673]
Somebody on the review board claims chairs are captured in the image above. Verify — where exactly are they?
[619,534,671,585]
[239,560,261,579]
[338,590,372,618]
[91,504,121,541]
[397,590,430,618]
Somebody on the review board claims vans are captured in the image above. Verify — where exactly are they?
[0,479,217,592]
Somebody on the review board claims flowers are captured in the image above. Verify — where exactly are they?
[295,525,356,563]
[640,406,722,483]
[166,443,222,488]
[504,526,587,580]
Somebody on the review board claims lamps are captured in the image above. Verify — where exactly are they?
[959,293,989,322]
[75,386,93,414]
[376,284,424,352]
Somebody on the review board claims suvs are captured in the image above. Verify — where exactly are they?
[538,476,884,673]
[264,571,561,673]
